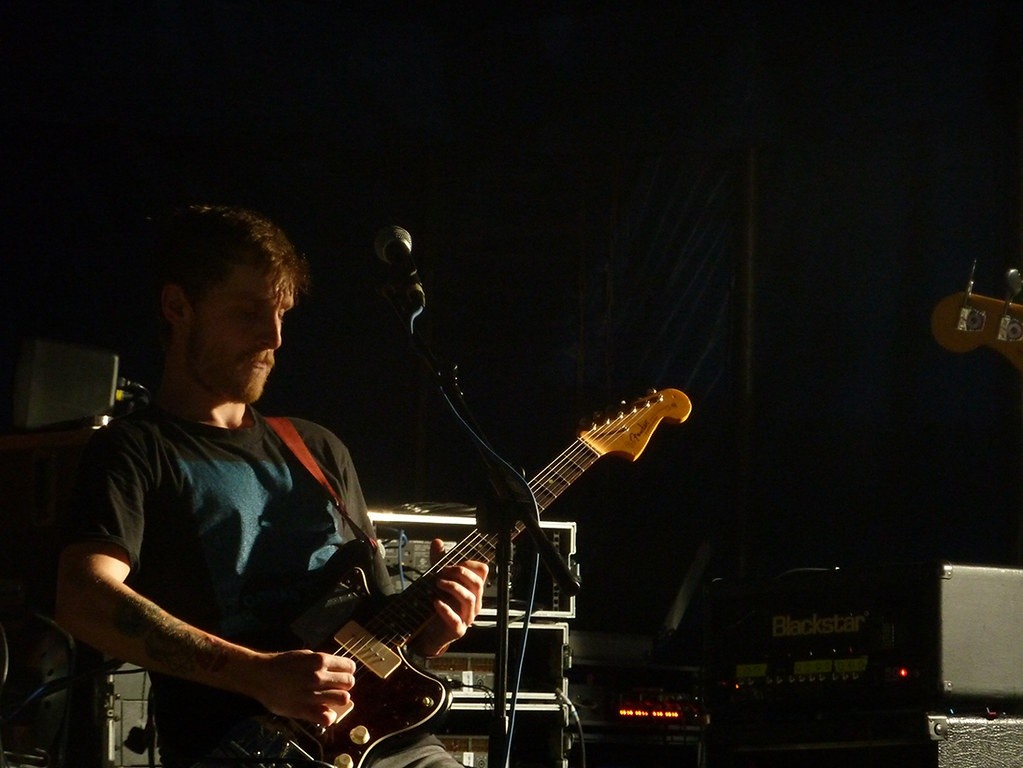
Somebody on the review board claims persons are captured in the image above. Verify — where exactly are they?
[47,208,487,766]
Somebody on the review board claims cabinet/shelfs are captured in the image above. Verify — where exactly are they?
[369,512,580,768]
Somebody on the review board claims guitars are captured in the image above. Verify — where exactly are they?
[189,386,693,768]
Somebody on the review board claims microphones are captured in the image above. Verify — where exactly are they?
[372,225,425,308]
